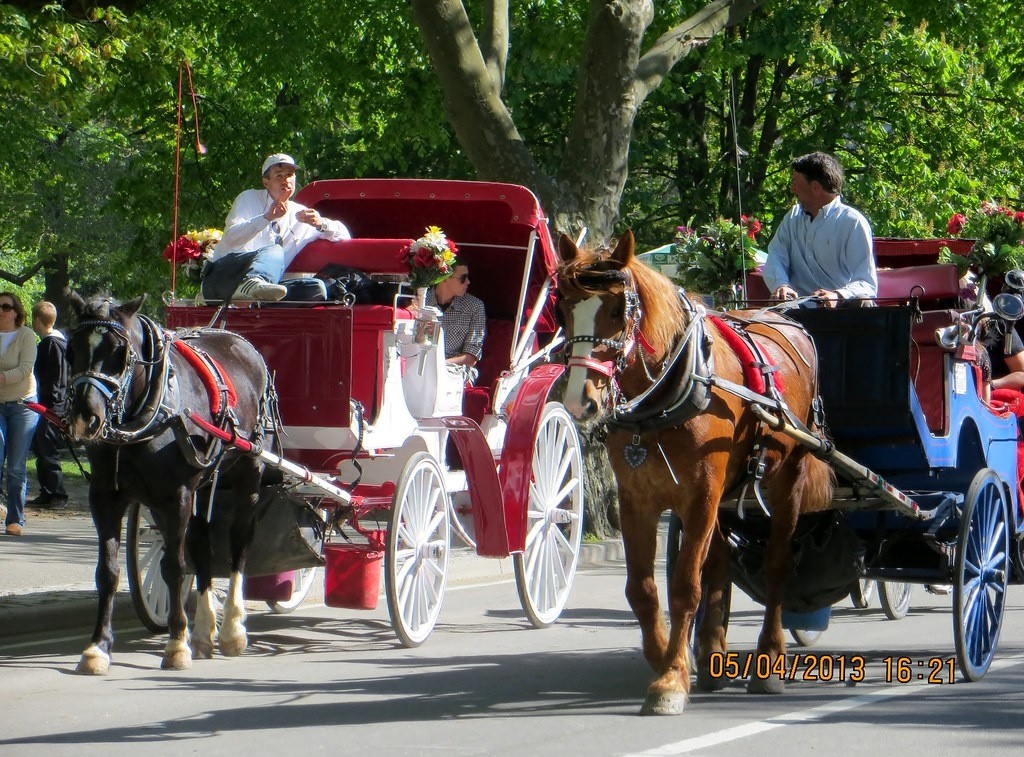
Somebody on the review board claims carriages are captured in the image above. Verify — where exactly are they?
[18,176,586,679]
[550,229,1024,719]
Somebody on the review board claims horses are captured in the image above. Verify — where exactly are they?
[51,281,280,673]
[548,224,832,718]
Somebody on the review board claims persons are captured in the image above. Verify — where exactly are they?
[412,257,485,387]
[956,267,1023,391]
[26,301,70,509]
[199,152,351,309]
[763,152,880,308]
[0,291,38,538]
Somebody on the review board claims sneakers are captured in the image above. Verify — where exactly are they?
[6,523,21,536]
[26,494,51,505]
[50,495,69,509]
[231,278,287,301]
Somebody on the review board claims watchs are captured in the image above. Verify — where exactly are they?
[316,219,327,232]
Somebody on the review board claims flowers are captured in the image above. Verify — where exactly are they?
[673,213,762,310]
[162,228,226,298]
[938,200,1023,281]
[399,225,458,292]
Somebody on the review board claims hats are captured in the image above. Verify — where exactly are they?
[262,153,301,176]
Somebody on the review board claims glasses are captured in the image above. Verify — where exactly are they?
[0,303,15,312]
[448,273,471,284]
